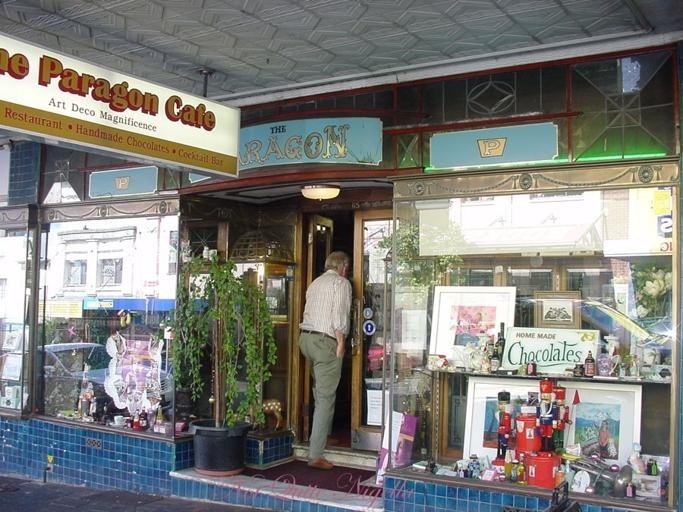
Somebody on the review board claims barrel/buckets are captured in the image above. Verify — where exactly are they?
[188,418,253,472]
[525,450,562,489]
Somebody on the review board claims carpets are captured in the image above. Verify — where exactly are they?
[240,460,381,497]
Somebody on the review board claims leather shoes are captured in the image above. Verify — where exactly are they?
[307,458,333,469]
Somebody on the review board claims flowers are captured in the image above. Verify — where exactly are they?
[609,262,673,319]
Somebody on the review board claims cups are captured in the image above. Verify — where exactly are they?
[113,416,123,424]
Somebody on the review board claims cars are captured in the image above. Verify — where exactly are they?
[516,295,671,369]
[0,340,176,427]
[368,341,384,377]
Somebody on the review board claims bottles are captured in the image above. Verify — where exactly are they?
[573,363,584,377]
[127,405,172,437]
[487,321,506,373]
[585,350,595,378]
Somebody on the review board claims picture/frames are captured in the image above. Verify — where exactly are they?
[425,285,673,466]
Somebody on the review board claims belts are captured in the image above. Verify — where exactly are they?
[300,329,337,341]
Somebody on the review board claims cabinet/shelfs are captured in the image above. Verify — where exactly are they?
[0,320,29,418]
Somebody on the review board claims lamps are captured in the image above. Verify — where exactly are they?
[301,185,341,202]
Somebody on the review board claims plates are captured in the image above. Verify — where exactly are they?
[109,422,125,428]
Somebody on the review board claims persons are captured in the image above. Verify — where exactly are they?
[295,251,354,470]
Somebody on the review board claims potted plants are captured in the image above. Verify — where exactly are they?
[153,257,277,476]
